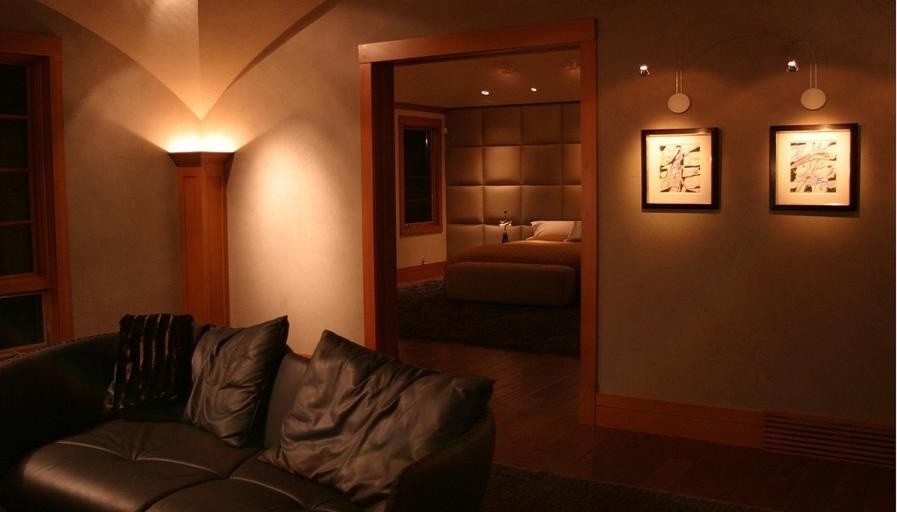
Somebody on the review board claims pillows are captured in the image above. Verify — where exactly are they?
[527,221,575,241]
[254,328,495,509]
[104,314,196,421]
[184,315,289,449]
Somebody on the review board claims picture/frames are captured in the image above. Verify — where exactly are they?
[768,122,861,212]
[640,126,720,211]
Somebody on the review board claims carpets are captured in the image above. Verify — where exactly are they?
[483,461,768,512]
[396,277,580,356]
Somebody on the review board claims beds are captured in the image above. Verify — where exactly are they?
[443,220,582,309]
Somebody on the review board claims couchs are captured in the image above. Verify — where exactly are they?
[0,329,496,512]
